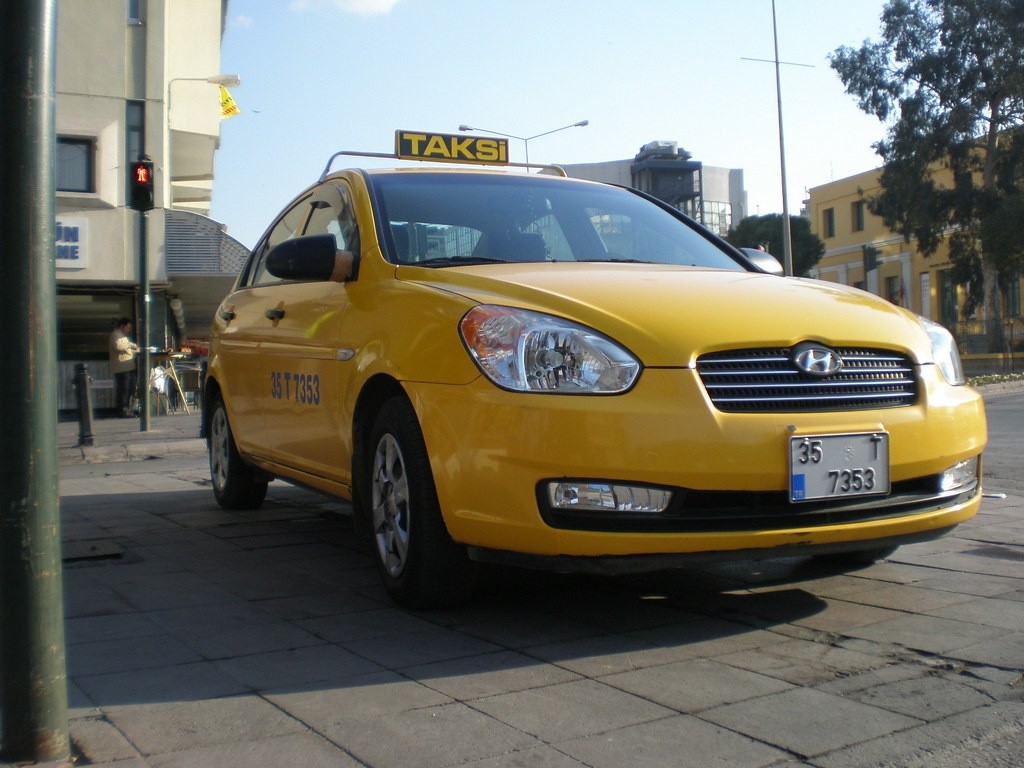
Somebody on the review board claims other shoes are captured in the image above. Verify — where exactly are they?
[118,411,137,418]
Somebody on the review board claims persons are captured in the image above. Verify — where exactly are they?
[109,317,141,418]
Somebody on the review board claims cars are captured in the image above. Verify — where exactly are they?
[197,132,989,618]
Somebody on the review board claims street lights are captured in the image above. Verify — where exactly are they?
[456,119,592,234]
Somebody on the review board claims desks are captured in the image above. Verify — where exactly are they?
[134,352,191,415]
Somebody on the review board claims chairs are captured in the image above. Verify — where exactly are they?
[491,230,548,263]
[392,226,409,264]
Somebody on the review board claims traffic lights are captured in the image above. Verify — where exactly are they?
[127,160,156,212]
